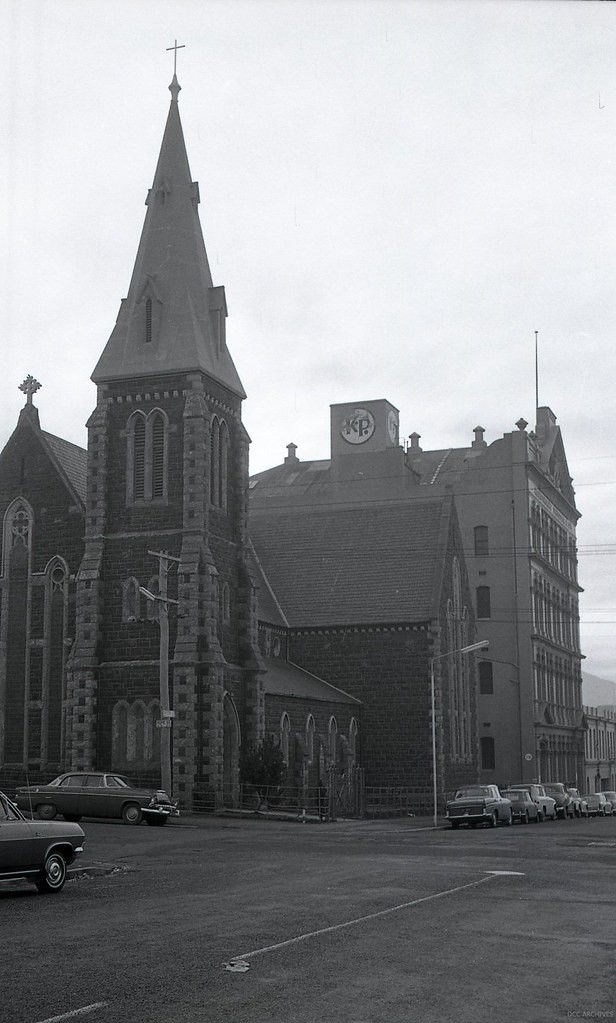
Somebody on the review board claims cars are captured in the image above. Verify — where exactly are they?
[444,784,513,829]
[567,787,590,819]
[16,771,180,826]
[540,783,576,820]
[601,791,616,816]
[0,791,85,897]
[580,792,612,817]
[511,783,557,822]
[500,790,540,824]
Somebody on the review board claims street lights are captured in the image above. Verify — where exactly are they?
[431,640,491,826]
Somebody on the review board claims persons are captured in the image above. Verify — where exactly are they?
[315,780,327,819]
